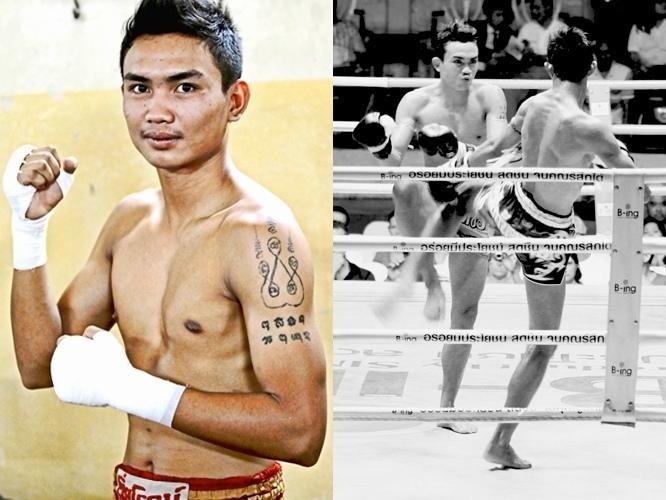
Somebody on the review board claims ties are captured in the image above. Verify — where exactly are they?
[492,31,498,50]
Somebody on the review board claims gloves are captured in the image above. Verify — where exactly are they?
[417,122,476,159]
[430,160,469,201]
[352,111,396,159]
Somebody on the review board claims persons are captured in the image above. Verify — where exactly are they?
[351,19,511,437]
[373,23,651,473]
[2,0,332,500]
[333,0,666,281]
